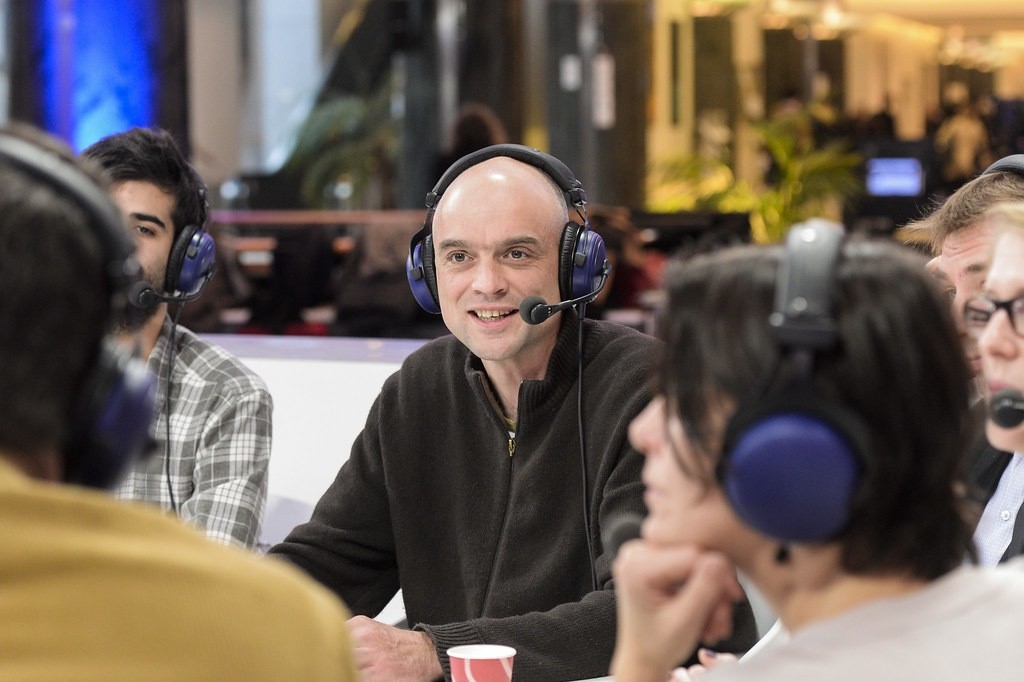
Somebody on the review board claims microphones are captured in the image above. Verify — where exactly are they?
[519,259,612,325]
[129,263,217,308]
[990,388,1024,428]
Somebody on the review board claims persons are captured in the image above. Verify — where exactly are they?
[450,106,505,154]
[266,144,758,682]
[0,123,353,682]
[929,87,1024,192]
[82,125,274,550]
[896,154,1024,567]
[610,219,1024,682]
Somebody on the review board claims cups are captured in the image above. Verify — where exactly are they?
[445,643,518,682]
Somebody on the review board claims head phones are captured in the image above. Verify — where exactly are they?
[407,144,606,314]
[0,133,161,490]
[716,215,869,544]
[163,164,215,298]
